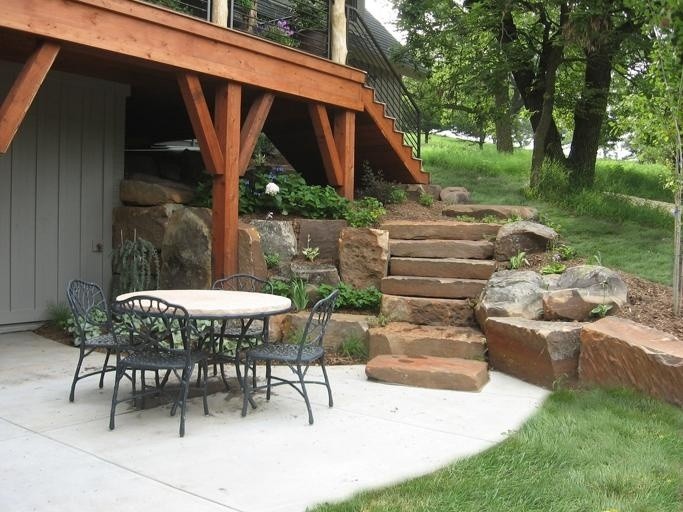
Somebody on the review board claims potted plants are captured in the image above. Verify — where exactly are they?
[293,0,328,58]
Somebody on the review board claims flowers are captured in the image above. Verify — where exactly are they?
[259,20,293,47]
